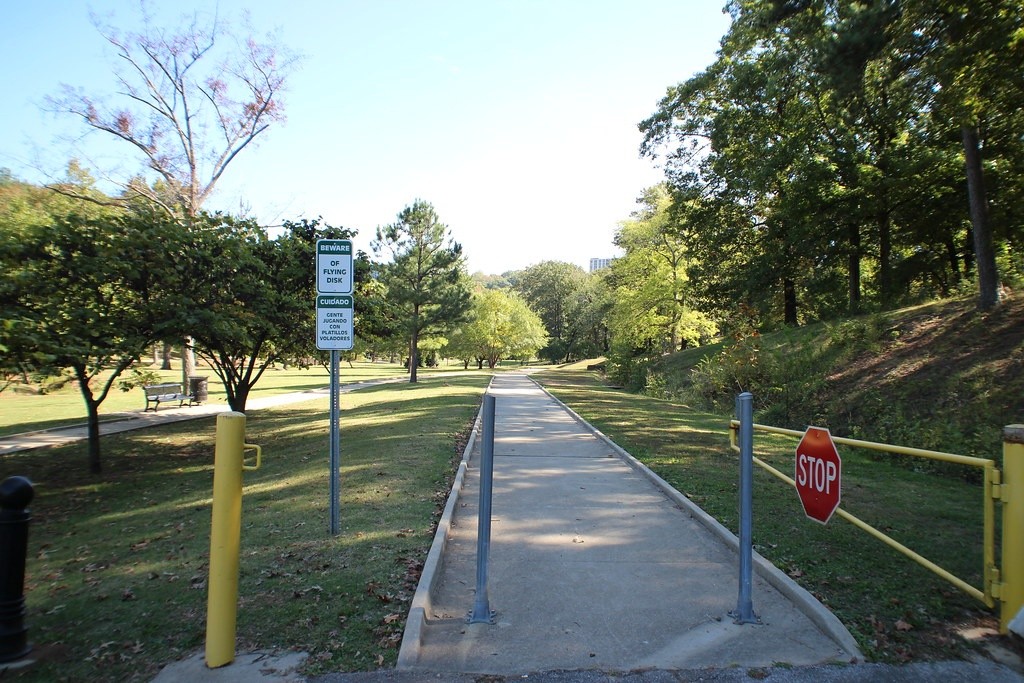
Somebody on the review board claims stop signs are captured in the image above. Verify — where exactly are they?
[794,425,842,526]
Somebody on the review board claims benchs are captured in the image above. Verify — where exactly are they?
[142,384,195,412]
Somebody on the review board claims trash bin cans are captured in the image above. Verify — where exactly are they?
[189,376,208,401]
